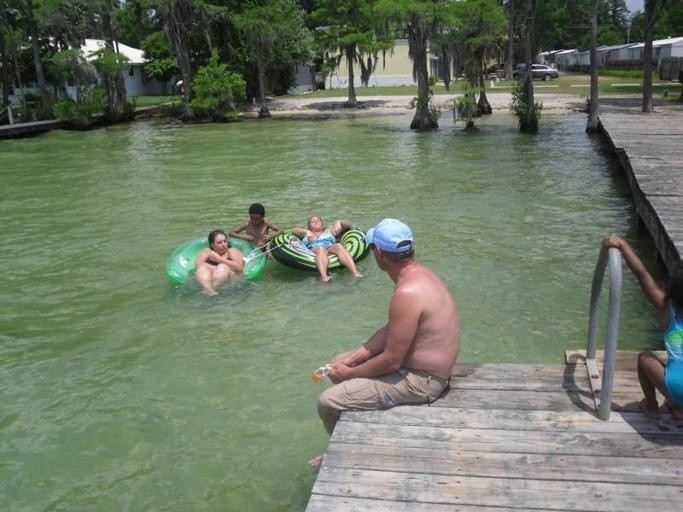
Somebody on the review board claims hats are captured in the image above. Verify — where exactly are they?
[365,217,415,254]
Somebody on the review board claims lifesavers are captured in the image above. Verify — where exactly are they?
[168,236,267,292]
[270,228,371,270]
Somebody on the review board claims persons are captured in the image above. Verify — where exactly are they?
[228,203,283,255]
[602,233,683,423]
[194,228,244,296]
[306,218,461,467]
[291,216,364,282]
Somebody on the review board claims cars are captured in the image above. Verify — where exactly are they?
[481,61,516,80]
[511,64,559,82]
